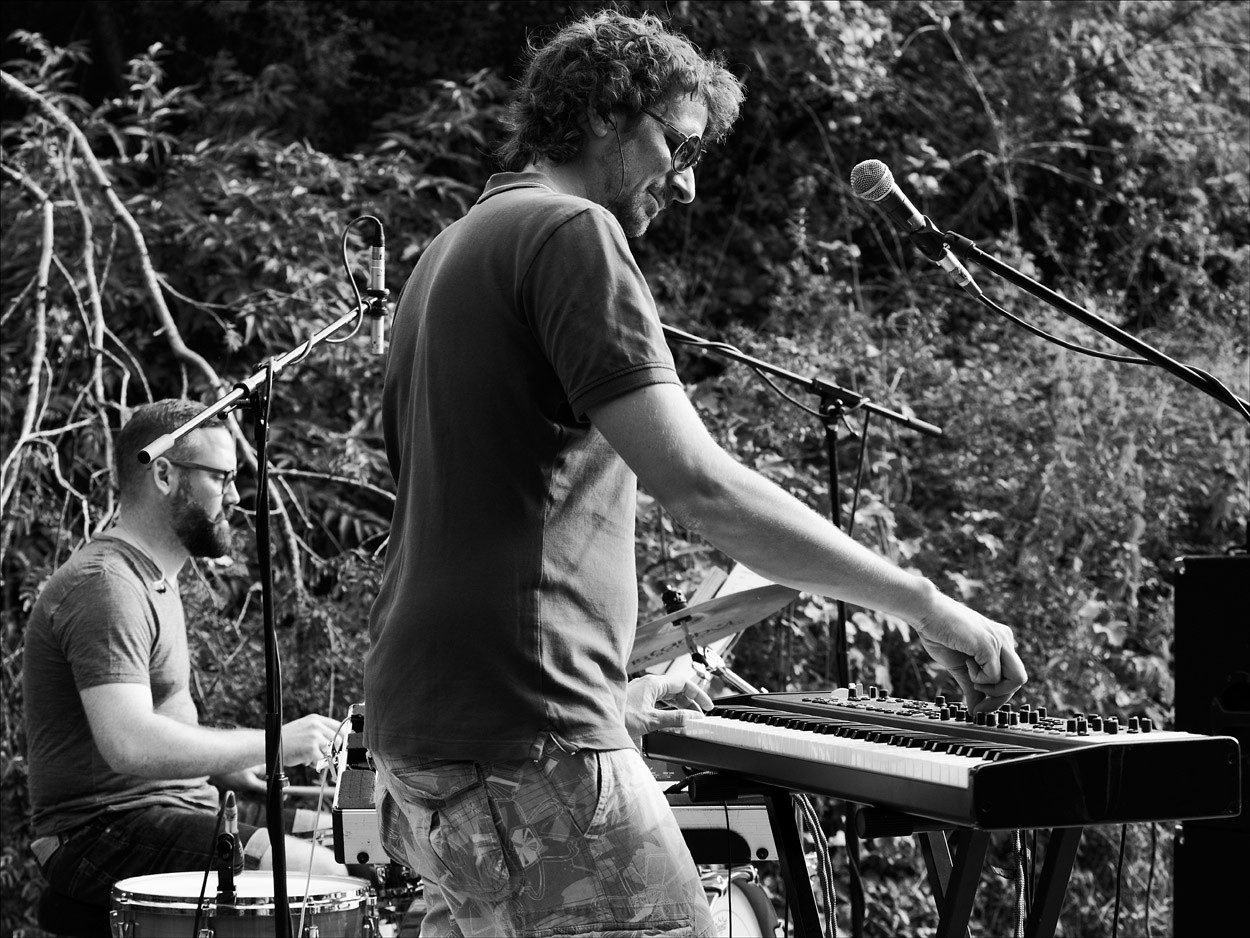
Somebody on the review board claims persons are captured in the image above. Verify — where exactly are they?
[21,397,350,937]
[361,10,1030,938]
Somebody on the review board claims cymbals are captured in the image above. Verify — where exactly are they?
[625,583,804,676]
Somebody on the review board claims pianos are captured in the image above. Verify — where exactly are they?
[641,684,1242,938]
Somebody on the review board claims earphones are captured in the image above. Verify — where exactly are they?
[596,102,610,122]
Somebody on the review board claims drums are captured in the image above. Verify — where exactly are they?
[109,869,376,938]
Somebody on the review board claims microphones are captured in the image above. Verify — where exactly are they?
[850,159,982,299]
[224,792,244,876]
[372,224,384,355]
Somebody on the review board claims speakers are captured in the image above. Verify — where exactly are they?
[1170,554,1250,938]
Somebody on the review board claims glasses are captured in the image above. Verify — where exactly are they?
[146,460,238,494]
[640,104,702,175]
